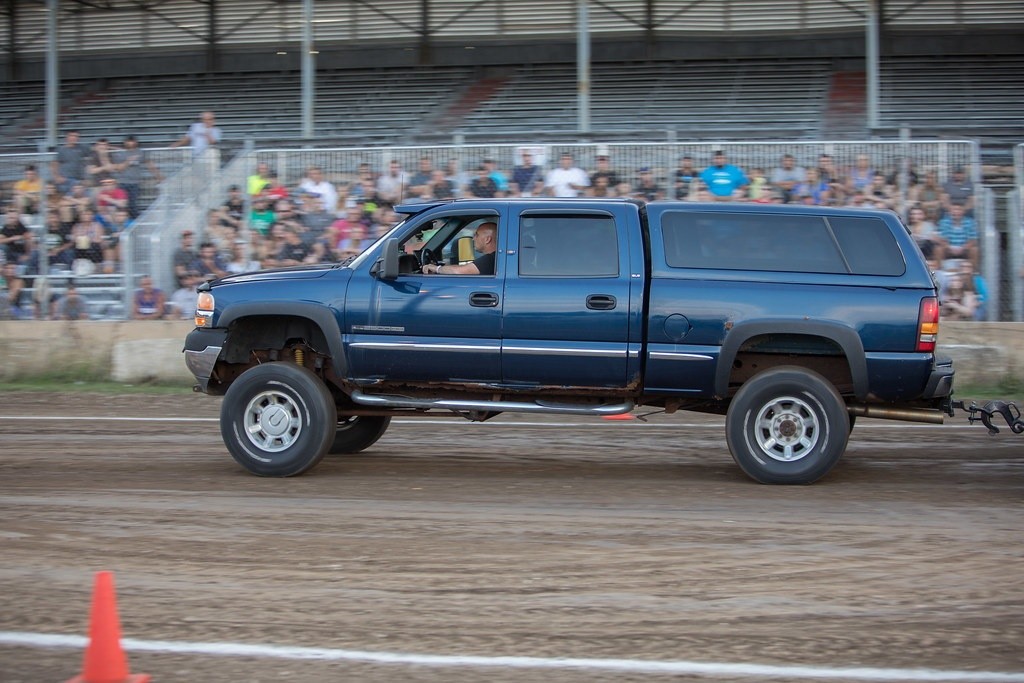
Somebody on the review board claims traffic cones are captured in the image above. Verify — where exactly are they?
[60,565,151,683]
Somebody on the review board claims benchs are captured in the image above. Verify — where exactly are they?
[17,192,195,321]
[0,57,1024,146]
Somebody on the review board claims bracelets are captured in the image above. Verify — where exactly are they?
[436,265,441,274]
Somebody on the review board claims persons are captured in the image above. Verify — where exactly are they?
[422,222,497,275]
[0,110,985,321]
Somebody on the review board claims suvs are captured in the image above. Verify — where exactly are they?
[181,197,957,487]
